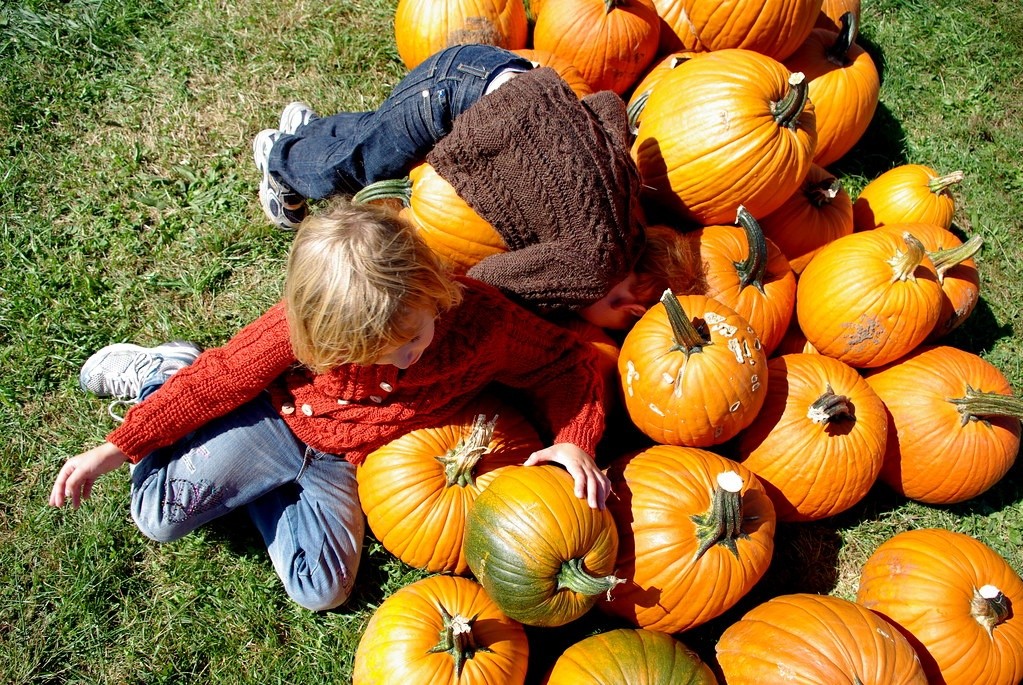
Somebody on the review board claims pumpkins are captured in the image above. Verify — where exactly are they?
[350,0,1023,685]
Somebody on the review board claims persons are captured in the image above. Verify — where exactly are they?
[253,43,701,332]
[50,206,610,610]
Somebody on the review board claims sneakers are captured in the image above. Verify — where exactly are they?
[280,102,319,135]
[252,129,309,230]
[81,341,205,423]
